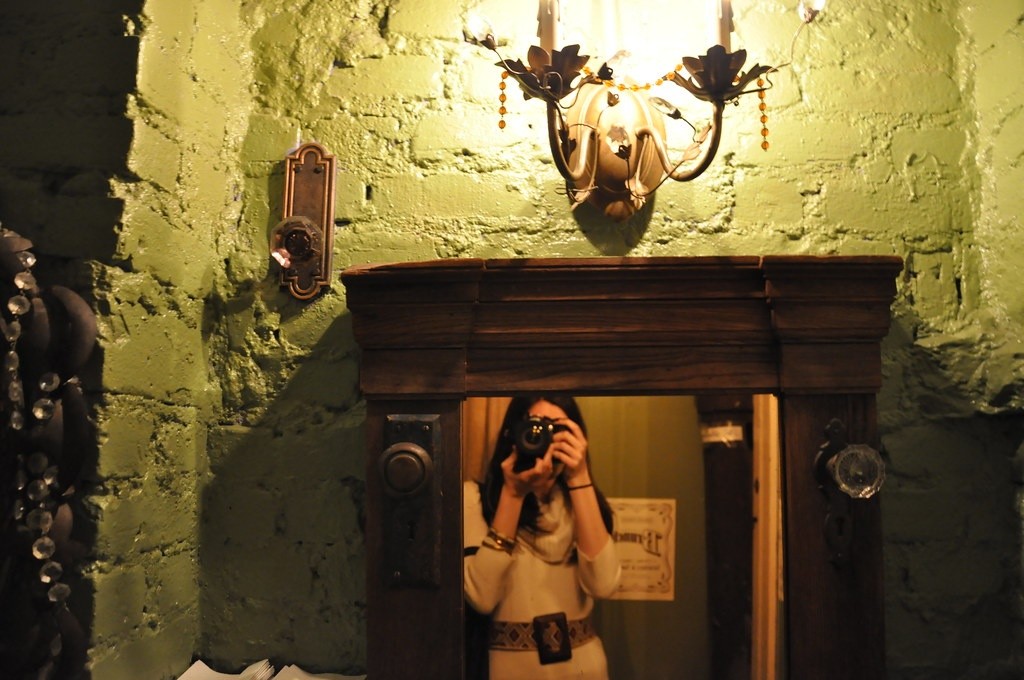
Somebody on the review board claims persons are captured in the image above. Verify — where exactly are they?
[463,394,622,680]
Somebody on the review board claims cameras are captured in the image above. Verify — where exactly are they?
[511,415,569,459]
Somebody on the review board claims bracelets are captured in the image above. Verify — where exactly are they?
[567,482,593,490]
[482,530,515,552]
